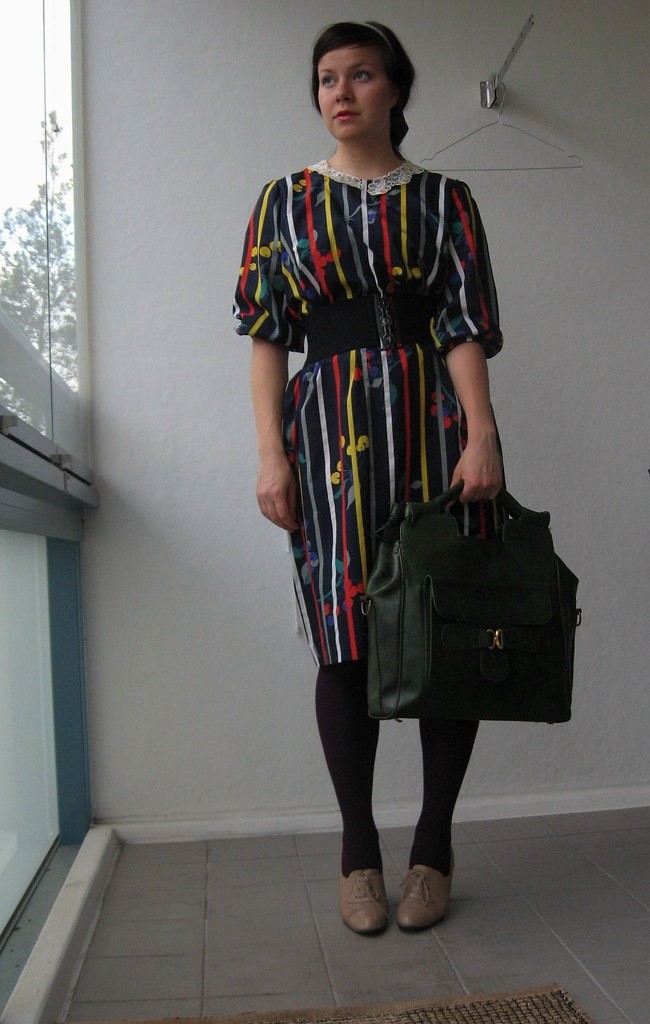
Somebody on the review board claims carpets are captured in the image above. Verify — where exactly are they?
[57,980,592,1024]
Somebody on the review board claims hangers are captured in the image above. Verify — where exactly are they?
[418,82,584,171]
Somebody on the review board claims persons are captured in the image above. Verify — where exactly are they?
[230,21,503,932]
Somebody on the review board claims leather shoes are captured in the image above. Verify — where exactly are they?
[338,848,390,935]
[396,848,455,930]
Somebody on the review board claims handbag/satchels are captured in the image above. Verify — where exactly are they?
[359,479,581,724]
